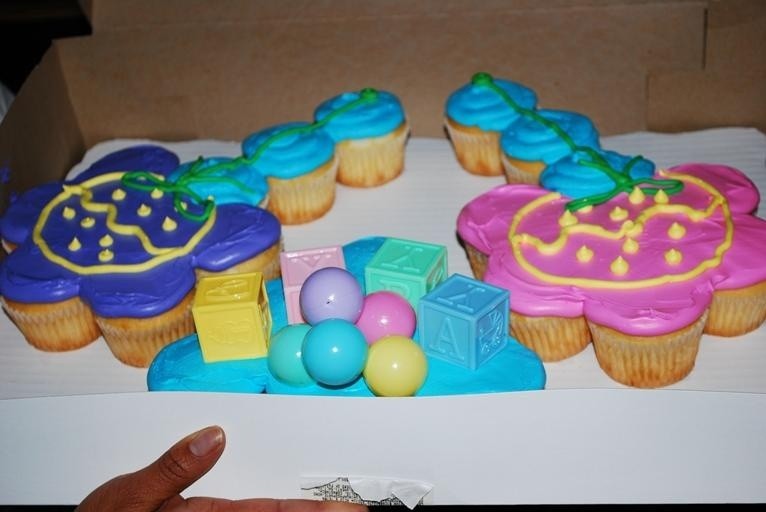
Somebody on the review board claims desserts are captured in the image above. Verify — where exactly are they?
[0,71,765,396]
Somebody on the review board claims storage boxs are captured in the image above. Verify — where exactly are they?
[0,0,766,509]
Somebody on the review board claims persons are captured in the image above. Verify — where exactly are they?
[74,426,367,511]
